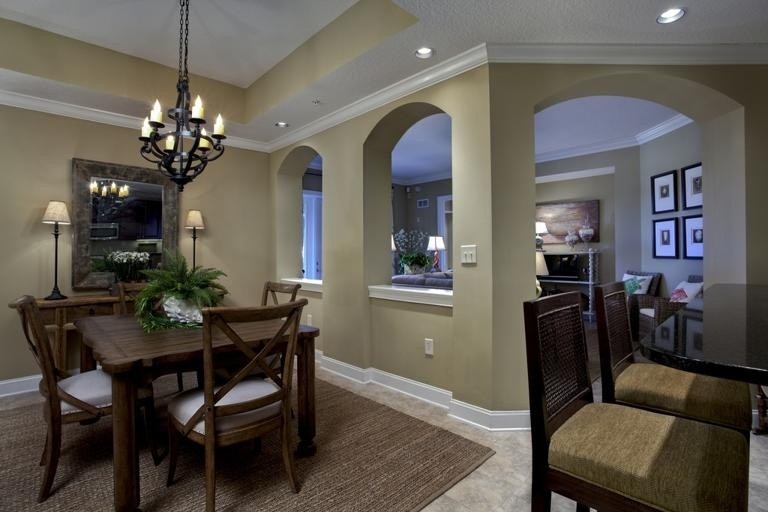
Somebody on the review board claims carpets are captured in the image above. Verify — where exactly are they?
[1,361,496,512]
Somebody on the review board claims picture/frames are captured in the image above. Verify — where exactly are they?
[680,160,703,211]
[681,214,703,260]
[650,169,678,215]
[651,216,678,259]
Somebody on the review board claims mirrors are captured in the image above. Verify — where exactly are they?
[68,156,180,292]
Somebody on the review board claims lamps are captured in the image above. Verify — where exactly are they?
[426,236,447,272]
[39,200,71,299]
[184,209,205,270]
[535,221,549,248]
[535,252,550,298]
[90,178,131,220]
[137,0,228,192]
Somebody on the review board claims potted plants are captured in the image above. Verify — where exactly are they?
[402,253,427,275]
[133,245,231,331]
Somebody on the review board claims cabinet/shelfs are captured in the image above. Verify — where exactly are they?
[537,249,599,324]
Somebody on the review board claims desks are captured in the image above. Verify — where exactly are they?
[73,304,319,511]
[639,282,766,437]
[33,290,233,399]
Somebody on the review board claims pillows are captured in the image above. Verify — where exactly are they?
[621,273,652,294]
[627,269,662,296]
[669,280,703,303]
[687,275,703,283]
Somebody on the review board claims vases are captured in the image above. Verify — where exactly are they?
[565,231,579,251]
[579,225,594,251]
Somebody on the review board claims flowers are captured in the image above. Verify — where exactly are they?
[105,247,151,283]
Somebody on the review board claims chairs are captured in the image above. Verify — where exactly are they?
[594,280,753,432]
[117,279,184,393]
[196,280,302,415]
[524,292,751,512]
[7,293,163,504]
[165,297,309,512]
